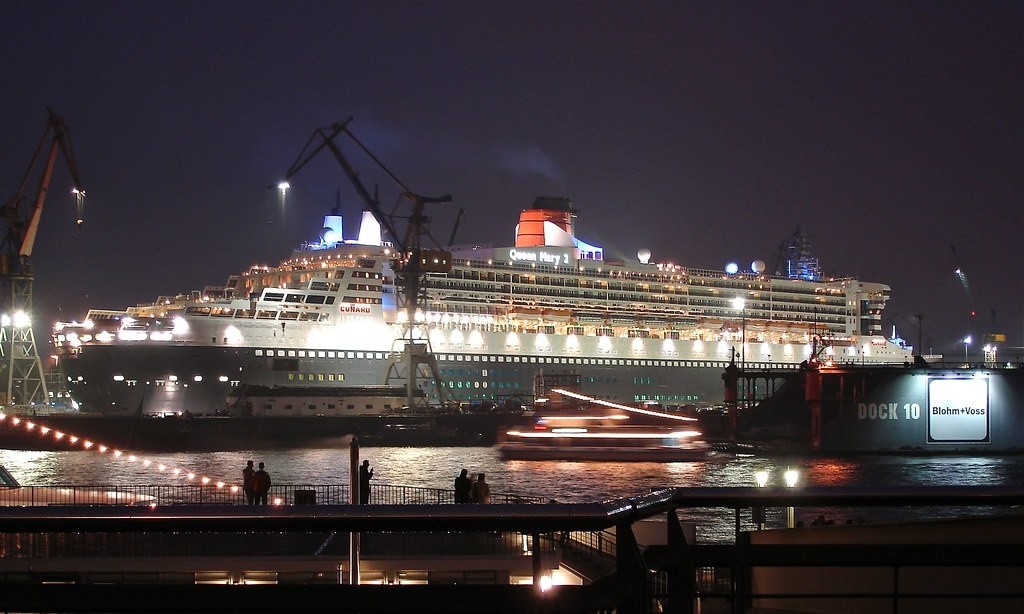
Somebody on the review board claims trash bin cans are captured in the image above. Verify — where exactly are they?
[294,490,316,505]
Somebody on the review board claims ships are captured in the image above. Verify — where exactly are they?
[56,196,917,414]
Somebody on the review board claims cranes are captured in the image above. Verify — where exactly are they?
[283,114,460,409]
[0,112,87,415]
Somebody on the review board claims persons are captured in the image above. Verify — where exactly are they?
[360,460,374,505]
[454,469,491,504]
[243,460,272,505]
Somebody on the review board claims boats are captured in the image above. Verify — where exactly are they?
[509,307,542,321]
[542,308,569,322]
[500,401,708,463]
